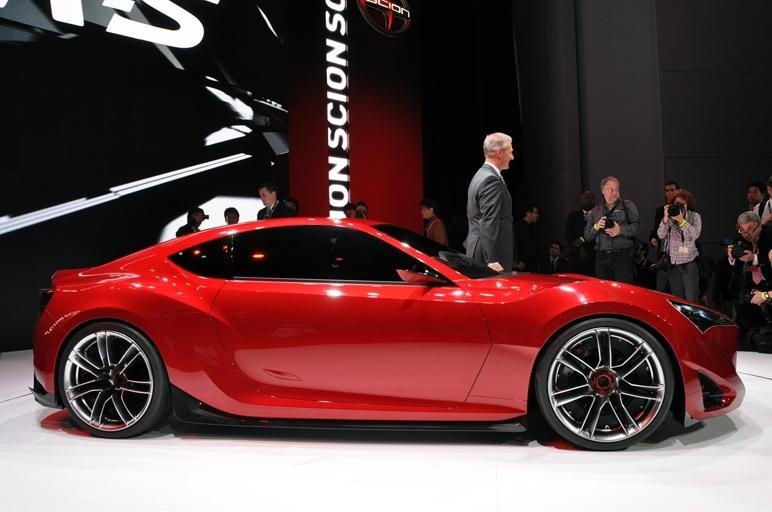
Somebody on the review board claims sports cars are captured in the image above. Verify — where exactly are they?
[27,218,750,451]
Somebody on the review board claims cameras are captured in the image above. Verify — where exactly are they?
[355,212,363,219]
[731,241,747,258]
[605,219,614,228]
[668,203,683,216]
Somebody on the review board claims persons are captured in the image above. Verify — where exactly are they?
[176,207,205,238]
[343,201,368,221]
[513,204,539,271]
[257,183,297,220]
[224,208,240,224]
[420,197,448,246]
[637,182,771,354]
[284,197,297,215]
[541,176,640,284]
[465,133,515,275]
